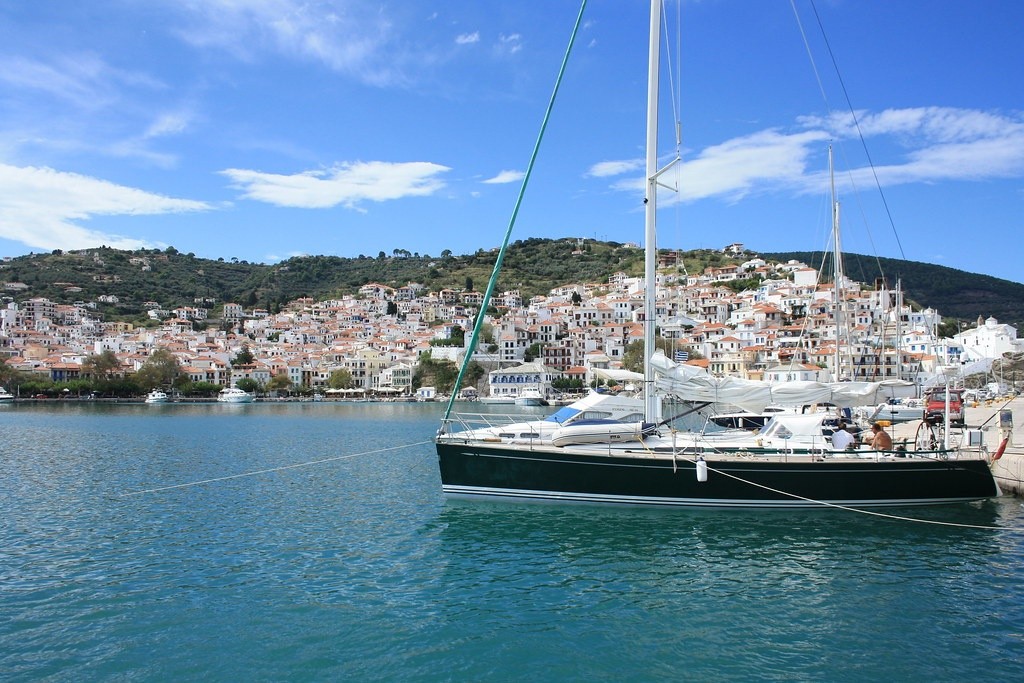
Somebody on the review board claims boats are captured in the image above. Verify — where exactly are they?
[216,387,256,403]
[145,388,169,403]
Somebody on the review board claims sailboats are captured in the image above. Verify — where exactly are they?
[433,0,1003,505]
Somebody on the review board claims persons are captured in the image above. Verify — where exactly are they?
[865,423,892,455]
[831,423,855,450]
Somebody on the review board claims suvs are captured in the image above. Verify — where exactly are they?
[924,386,966,428]
